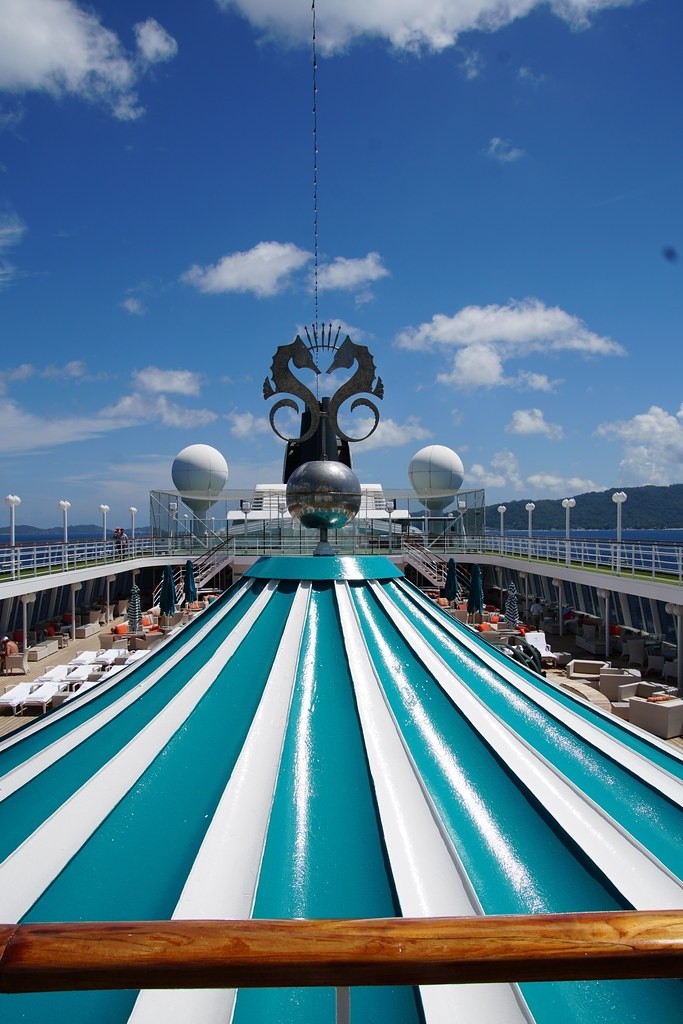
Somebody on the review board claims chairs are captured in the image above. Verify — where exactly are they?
[0,597,207,717]
[422,589,683,740]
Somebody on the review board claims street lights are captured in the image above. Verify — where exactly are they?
[211,516,216,535]
[98,504,110,565]
[4,494,22,581]
[384,501,394,554]
[561,498,577,568]
[58,499,72,572]
[612,491,627,578]
[457,501,467,552]
[524,502,536,562]
[184,513,189,538]
[169,503,178,549]
[128,506,137,560]
[498,506,507,558]
[278,505,287,555]
[448,513,453,535]
[241,501,252,553]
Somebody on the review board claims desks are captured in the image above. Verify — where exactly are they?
[628,697,682,736]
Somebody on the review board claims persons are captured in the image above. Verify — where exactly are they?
[58,612,70,627]
[577,614,590,627]
[97,595,107,605]
[0,636,17,673]
[114,528,128,559]
[531,598,543,630]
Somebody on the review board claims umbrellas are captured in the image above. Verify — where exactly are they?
[504,582,519,631]
[183,560,197,611]
[468,565,483,623]
[160,566,177,628]
[444,558,458,608]
[127,585,142,634]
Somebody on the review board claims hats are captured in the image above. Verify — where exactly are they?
[1,636,8,641]
